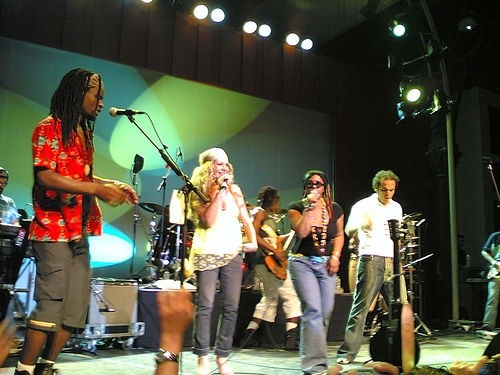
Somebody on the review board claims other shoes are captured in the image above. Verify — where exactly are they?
[336,357,350,364]
[34,362,57,375]
[476,325,492,331]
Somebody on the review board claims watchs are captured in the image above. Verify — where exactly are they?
[155,348,179,364]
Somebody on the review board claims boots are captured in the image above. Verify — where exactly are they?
[238,321,261,348]
[284,322,300,351]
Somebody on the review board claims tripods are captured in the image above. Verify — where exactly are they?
[131,148,180,282]
[403,238,432,338]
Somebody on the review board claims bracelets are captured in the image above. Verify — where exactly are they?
[331,251,341,261]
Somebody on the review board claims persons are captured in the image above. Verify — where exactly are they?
[154,291,194,375]
[0,68,139,375]
[288,171,345,375]
[336,171,408,364]
[451,332,500,375]
[240,186,303,351]
[481,232,500,332]
[328,362,454,375]
[189,148,257,375]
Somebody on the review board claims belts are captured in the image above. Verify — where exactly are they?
[289,255,330,263]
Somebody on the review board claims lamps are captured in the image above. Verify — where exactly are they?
[387,17,410,39]
[399,80,423,103]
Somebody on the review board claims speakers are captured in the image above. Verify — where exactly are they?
[326,292,354,342]
[136,287,197,348]
[232,289,265,348]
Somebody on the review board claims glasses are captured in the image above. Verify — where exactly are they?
[305,181,325,188]
[0,183,8,186]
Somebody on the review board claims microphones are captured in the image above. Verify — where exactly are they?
[179,146,184,163]
[221,178,228,190]
[109,107,145,118]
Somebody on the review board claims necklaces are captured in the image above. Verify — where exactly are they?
[302,198,329,252]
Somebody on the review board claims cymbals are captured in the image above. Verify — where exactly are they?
[139,202,168,215]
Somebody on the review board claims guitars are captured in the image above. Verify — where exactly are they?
[263,230,296,281]
[369,219,421,371]
